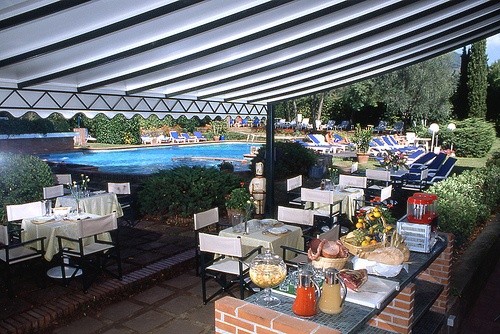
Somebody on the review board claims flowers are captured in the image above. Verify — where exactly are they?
[226,184,258,216]
[67,174,90,199]
[384,149,407,167]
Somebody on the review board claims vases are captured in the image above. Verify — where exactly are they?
[244,216,248,234]
[76,200,80,214]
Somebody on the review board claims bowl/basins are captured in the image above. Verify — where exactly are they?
[51,207,72,215]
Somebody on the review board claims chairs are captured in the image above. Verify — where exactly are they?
[141,117,458,304]
[0,174,138,294]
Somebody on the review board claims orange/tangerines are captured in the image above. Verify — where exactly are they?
[356,208,391,246]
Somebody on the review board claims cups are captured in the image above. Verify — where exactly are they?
[42,200,52,217]
[324,179,330,190]
[232,214,243,232]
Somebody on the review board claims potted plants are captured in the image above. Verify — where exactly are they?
[351,123,373,164]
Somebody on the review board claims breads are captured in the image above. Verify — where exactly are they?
[360,246,405,264]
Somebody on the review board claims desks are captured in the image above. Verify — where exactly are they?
[55,191,124,220]
[21,211,111,278]
[304,185,364,234]
[374,167,409,178]
[214,219,305,293]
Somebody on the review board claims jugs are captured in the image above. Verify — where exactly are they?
[291,260,322,318]
[319,262,347,314]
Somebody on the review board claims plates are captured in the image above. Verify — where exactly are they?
[345,188,359,192]
[267,227,288,234]
[260,218,279,225]
